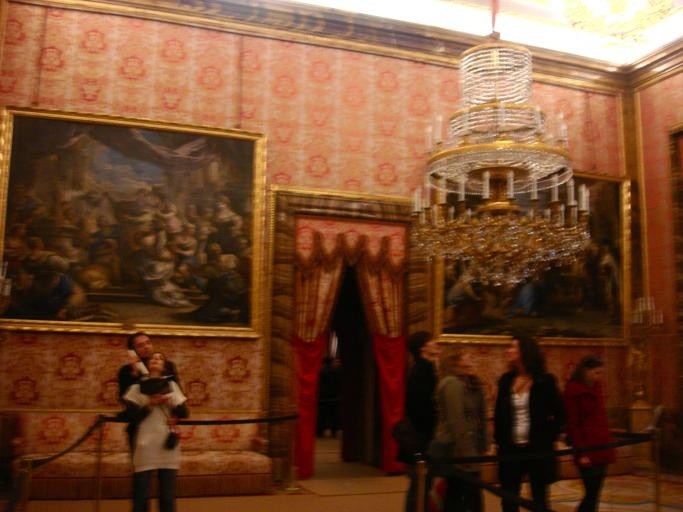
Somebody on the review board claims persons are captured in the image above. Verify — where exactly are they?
[316,353,347,439]
[121,353,190,512]
[118,332,184,453]
[441,243,621,336]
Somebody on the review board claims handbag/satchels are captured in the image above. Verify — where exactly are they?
[165,432,179,450]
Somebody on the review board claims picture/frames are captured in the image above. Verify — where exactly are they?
[432,163,633,349]
[0,105,268,340]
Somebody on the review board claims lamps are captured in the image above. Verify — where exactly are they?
[409,0,591,288]
